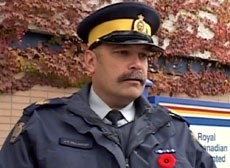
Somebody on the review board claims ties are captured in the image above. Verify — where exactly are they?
[105,110,124,128]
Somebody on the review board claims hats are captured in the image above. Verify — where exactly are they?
[77,3,165,52]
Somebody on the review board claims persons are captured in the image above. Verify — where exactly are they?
[0,3,217,168]
